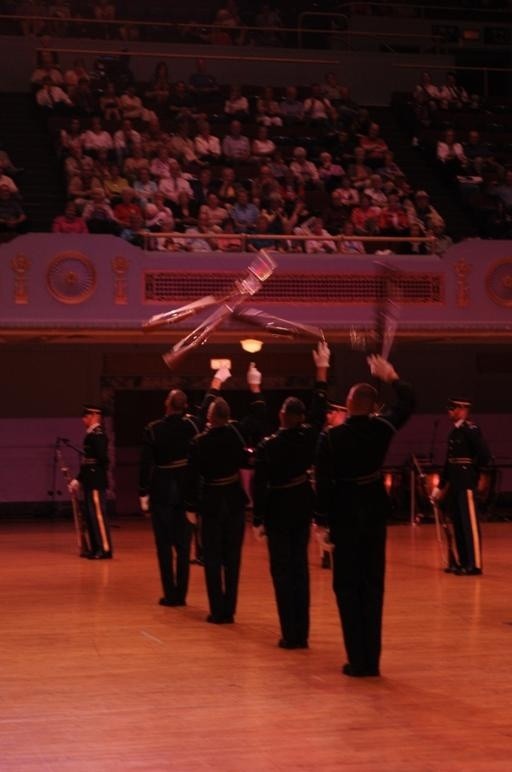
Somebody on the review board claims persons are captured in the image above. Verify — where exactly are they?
[188,359,263,622]
[251,342,337,650]
[141,363,233,606]
[429,395,491,572]
[314,350,419,678]
[67,404,115,560]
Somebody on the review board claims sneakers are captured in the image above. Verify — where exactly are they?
[86,549,113,559]
[158,596,382,677]
[457,565,481,575]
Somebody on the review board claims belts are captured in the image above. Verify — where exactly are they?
[202,472,240,487]
[152,459,189,470]
[445,457,476,465]
[80,458,97,465]
[333,470,382,486]
[266,473,308,489]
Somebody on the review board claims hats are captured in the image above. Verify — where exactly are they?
[449,398,471,410]
[80,404,104,418]
[328,398,347,412]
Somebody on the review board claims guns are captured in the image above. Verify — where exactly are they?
[57,449,91,558]
[412,454,459,572]
[140,249,324,369]
